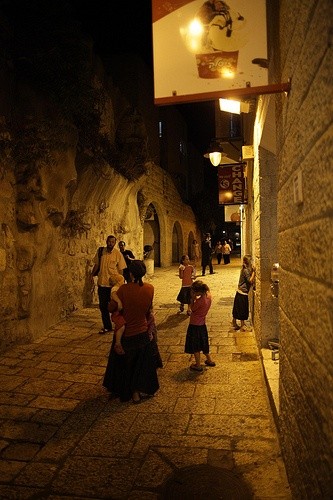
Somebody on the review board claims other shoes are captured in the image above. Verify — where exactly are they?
[204,359,216,367]
[99,328,112,333]
[180,304,185,311]
[131,392,141,404]
[190,363,203,371]
[114,343,124,355]
[232,325,239,330]
[187,310,192,316]
[241,324,251,332]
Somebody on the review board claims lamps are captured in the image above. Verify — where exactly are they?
[208,135,247,171]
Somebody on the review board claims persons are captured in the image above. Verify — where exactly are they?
[191,240,200,263]
[222,240,231,264]
[109,273,155,355]
[201,233,215,276]
[232,254,256,329]
[185,280,216,371]
[118,241,137,284]
[215,241,222,264]
[92,236,127,334]
[176,255,197,316]
[103,260,163,402]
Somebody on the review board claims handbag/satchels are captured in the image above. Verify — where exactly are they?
[91,247,104,277]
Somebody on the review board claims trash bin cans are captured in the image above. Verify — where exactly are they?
[144,259,154,275]
